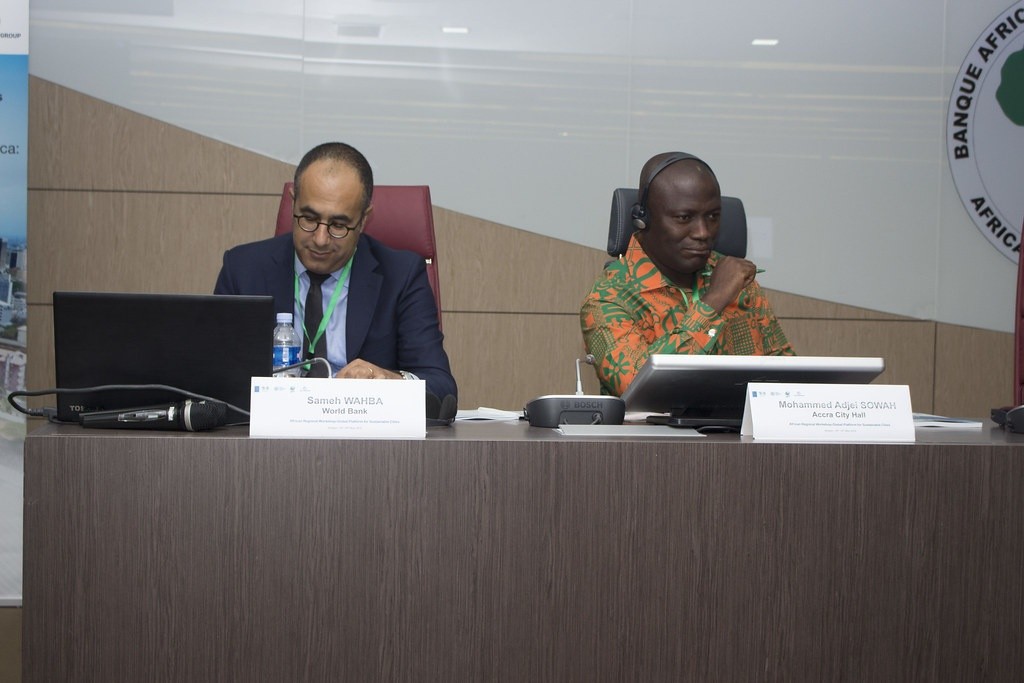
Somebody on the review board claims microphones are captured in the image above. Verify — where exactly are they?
[526,353,627,427]
[82,403,229,431]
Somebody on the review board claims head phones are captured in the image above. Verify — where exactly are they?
[631,154,714,232]
[991,404,1024,435]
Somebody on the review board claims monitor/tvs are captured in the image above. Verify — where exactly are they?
[620,354,885,426]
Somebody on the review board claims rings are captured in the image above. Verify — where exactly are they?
[367,366,375,379]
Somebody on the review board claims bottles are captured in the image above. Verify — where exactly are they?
[273,313,301,378]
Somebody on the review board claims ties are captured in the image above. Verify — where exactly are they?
[302,269,331,379]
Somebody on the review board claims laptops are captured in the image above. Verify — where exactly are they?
[53,292,274,423]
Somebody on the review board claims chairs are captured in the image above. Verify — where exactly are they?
[274,183,442,336]
[599,188,747,396]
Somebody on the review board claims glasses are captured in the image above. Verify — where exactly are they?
[290,196,366,240]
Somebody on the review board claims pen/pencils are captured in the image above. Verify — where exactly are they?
[700,268,766,277]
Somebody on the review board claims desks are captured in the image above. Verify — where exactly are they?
[23,413,1023,682]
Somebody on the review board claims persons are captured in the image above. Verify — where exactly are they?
[579,153,798,398]
[213,142,459,425]
